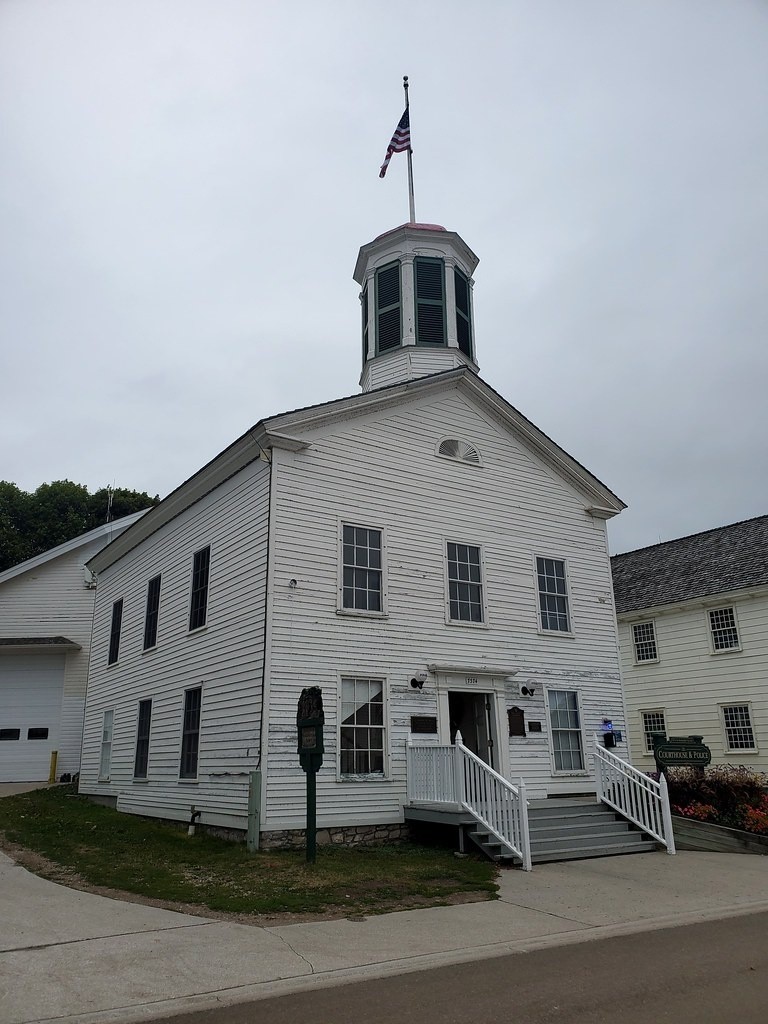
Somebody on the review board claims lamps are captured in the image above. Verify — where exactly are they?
[408,669,427,690]
[519,679,542,699]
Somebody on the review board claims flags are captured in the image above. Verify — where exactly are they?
[378,101,411,178]
